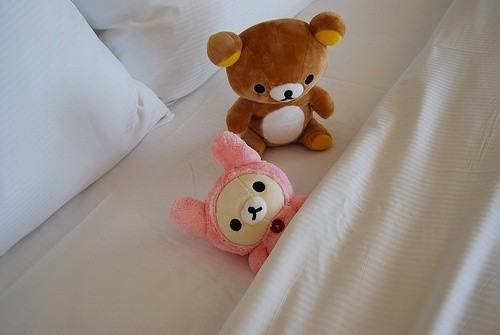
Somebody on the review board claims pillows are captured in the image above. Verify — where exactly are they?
[0,0,169,256]
[72,0,311,103]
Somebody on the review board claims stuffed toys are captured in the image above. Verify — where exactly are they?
[206,11,347,159]
[169,131,309,276]
